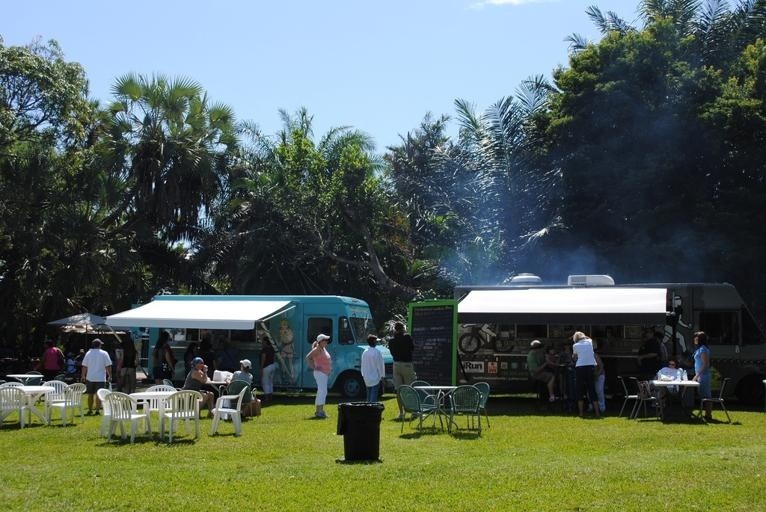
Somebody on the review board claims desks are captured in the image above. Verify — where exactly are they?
[6,374,44,385]
[129,391,175,445]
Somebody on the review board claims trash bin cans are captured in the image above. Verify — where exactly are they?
[338,401,385,463]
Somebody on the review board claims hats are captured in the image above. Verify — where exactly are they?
[240,359,252,369]
[92,339,104,344]
[317,334,330,342]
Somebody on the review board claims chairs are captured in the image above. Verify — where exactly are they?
[143,385,178,434]
[0,380,87,428]
[163,389,203,443]
[209,385,250,436]
[26,371,42,386]
[96,388,126,439]
[104,392,152,443]
[396,380,490,436]
[540,362,736,425]
[161,375,261,419]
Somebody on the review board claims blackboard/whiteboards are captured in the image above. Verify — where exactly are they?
[407,299,458,414]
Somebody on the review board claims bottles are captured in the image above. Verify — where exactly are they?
[676,369,687,381]
[657,369,661,381]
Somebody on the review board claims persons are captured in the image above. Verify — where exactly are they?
[23,339,136,417]
[153,318,294,418]
[388,322,419,421]
[526,331,606,418]
[359,334,384,405]
[657,332,712,423]
[304,334,331,419]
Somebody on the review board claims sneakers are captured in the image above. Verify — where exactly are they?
[316,411,328,417]
